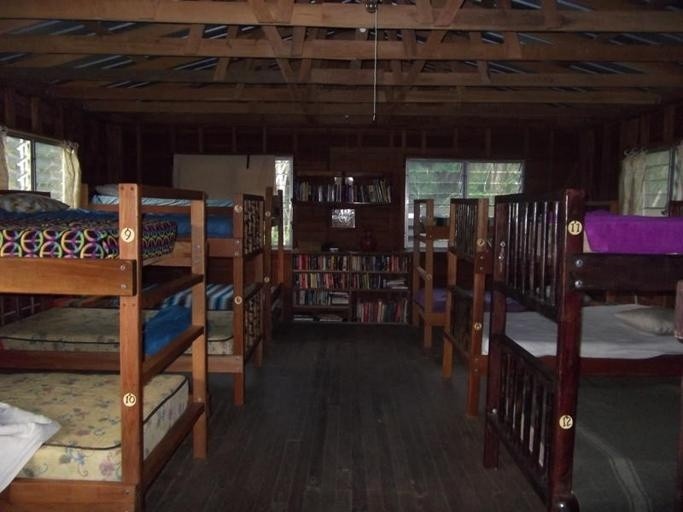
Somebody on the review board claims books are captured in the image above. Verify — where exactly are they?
[295,179,391,204]
[291,254,409,323]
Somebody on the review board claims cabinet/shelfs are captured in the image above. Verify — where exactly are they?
[288,249,409,330]
[289,173,395,210]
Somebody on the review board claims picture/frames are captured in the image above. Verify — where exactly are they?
[326,204,361,232]
